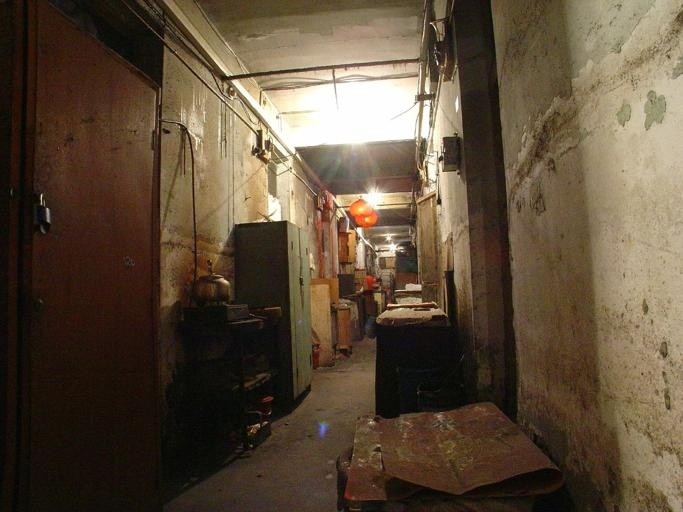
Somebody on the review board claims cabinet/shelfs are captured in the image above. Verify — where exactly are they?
[179,317,279,450]
[337,305,354,355]
[339,274,365,340]
[308,278,339,360]
[375,308,458,418]
[339,231,355,263]
[234,220,312,411]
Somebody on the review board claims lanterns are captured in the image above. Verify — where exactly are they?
[355,211,378,227]
[350,195,372,223]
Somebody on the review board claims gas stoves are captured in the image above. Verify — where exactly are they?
[181,304,251,323]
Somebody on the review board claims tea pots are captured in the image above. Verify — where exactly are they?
[188,259,231,307]
